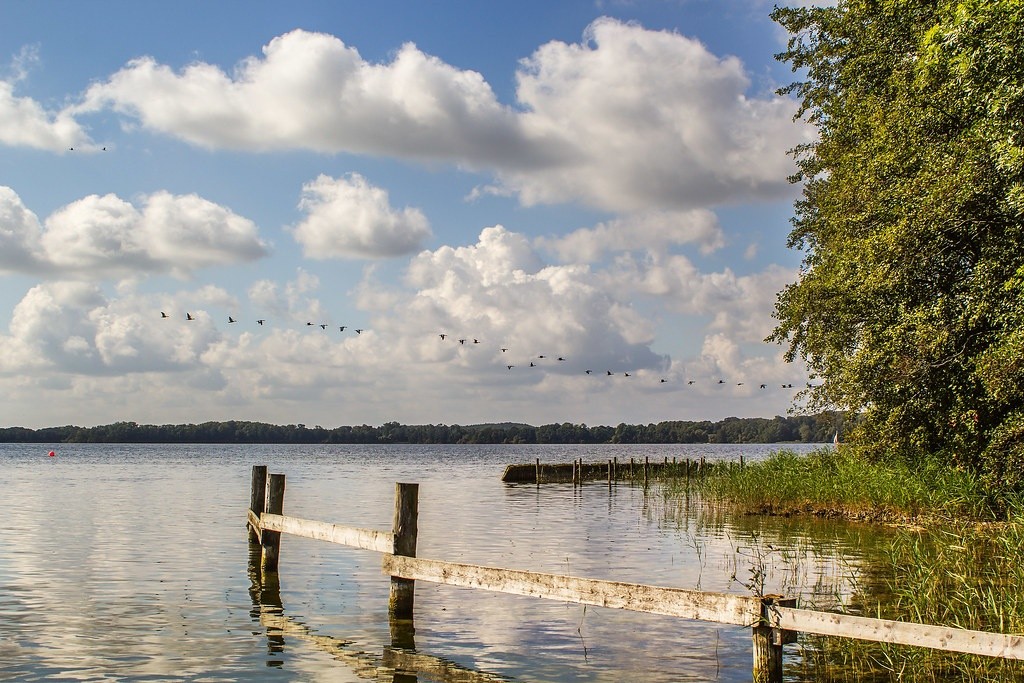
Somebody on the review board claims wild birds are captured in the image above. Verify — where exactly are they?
[70,147,106,151]
[161,312,816,389]
